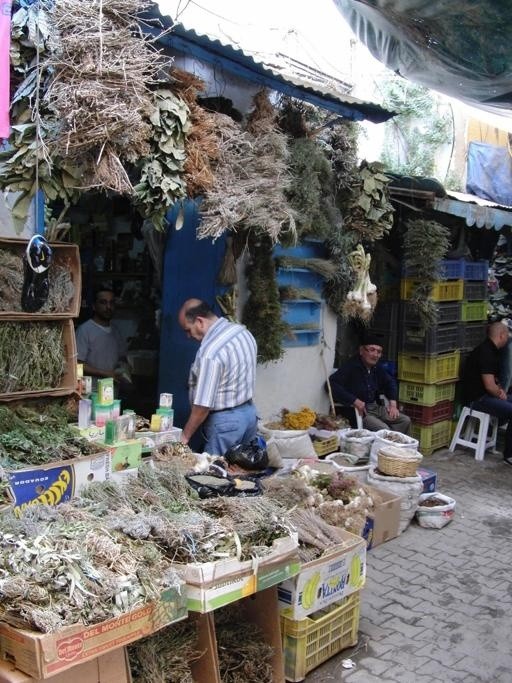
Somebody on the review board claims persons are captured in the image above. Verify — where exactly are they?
[325,335,411,435]
[75,288,134,415]
[456,314,512,467]
[177,297,262,467]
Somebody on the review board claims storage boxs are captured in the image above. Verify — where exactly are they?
[415,467,436,493]
[360,481,402,550]
[0,232,369,683]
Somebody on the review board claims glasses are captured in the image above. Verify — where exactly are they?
[363,345,383,355]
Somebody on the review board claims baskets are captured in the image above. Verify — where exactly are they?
[376,451,421,477]
[313,436,340,457]
[279,593,363,683]
[397,258,490,457]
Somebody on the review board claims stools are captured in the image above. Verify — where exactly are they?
[448,404,497,460]
